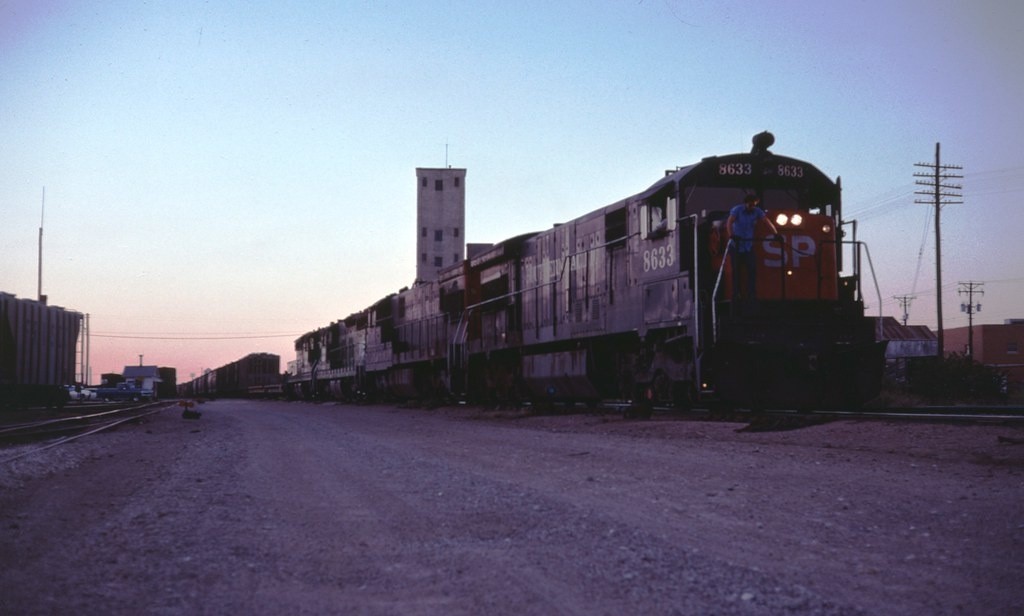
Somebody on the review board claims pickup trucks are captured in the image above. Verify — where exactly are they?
[98,382,150,402]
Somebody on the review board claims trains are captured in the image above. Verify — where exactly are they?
[295,131,888,420]
[176,352,280,395]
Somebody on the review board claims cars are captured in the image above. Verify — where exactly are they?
[66,384,98,402]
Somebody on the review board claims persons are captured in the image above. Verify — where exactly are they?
[727,194,778,302]
